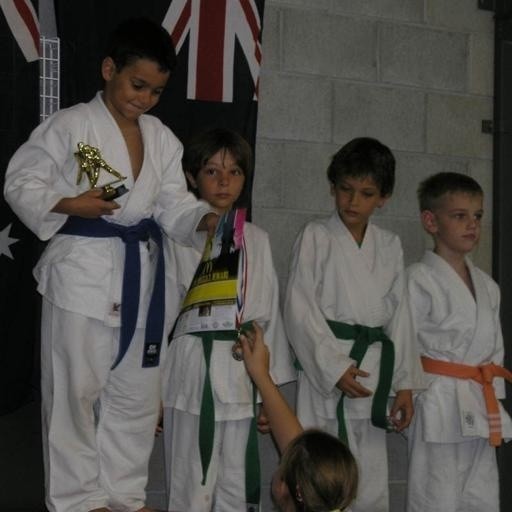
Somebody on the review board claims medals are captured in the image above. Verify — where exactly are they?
[232,340,244,361]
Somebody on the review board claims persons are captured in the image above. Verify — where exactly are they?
[156,128,297,512]
[403,173,512,512]
[238,322,359,512]
[283,137,430,512]
[3,16,219,512]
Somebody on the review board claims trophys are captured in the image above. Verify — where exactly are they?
[77,142,130,200]
